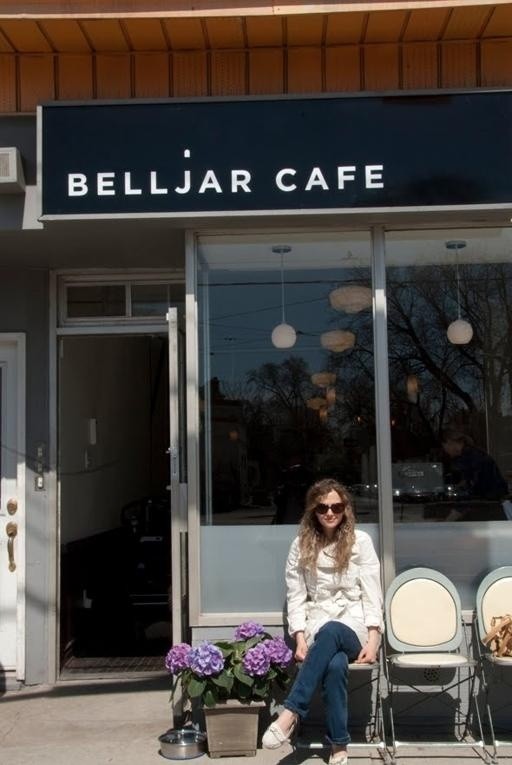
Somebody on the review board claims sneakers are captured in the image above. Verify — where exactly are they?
[261,718,299,750]
[328,744,348,765]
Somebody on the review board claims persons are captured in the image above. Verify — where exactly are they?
[273,420,509,522]
[261,480,382,765]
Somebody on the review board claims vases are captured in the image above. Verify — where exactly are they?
[195,697,266,760]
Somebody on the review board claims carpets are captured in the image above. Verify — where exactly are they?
[60,634,173,674]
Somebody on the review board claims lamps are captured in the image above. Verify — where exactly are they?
[311,363,339,391]
[327,279,373,314]
[318,322,357,355]
[443,238,474,344]
[310,393,329,413]
[270,243,298,349]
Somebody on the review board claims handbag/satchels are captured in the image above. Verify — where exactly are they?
[482,614,512,659]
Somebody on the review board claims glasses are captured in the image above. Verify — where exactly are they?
[313,502,347,515]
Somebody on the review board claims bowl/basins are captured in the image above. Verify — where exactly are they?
[157,731,209,760]
[354,481,469,504]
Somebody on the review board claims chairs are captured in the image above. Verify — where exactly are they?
[471,564,512,764]
[293,593,389,765]
[380,565,488,764]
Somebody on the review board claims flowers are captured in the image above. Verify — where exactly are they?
[162,621,298,713]
